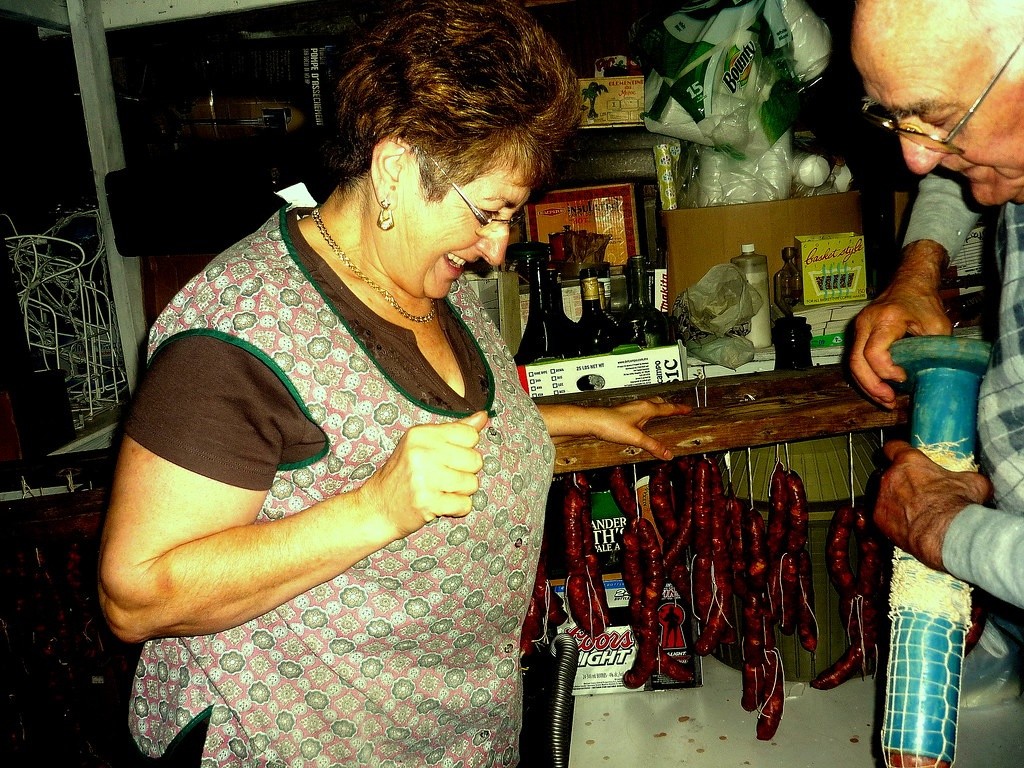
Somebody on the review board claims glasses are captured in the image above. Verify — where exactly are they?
[860,38,1024,154]
[421,148,526,239]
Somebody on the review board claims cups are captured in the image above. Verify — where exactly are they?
[505,224,612,284]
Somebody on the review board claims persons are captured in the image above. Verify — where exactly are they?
[850,0,1024,608]
[97,2,690,768]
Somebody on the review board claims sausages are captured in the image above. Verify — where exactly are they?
[524,452,987,741]
[888,754,949,768]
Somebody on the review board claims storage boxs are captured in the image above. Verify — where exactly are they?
[535,575,701,695]
[518,345,685,398]
[575,75,647,126]
[658,187,862,313]
[796,232,868,305]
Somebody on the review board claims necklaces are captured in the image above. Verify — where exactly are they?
[312,208,436,324]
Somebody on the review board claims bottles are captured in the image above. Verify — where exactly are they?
[770,312,812,370]
[772,247,802,313]
[514,239,668,365]
[731,243,771,349]
